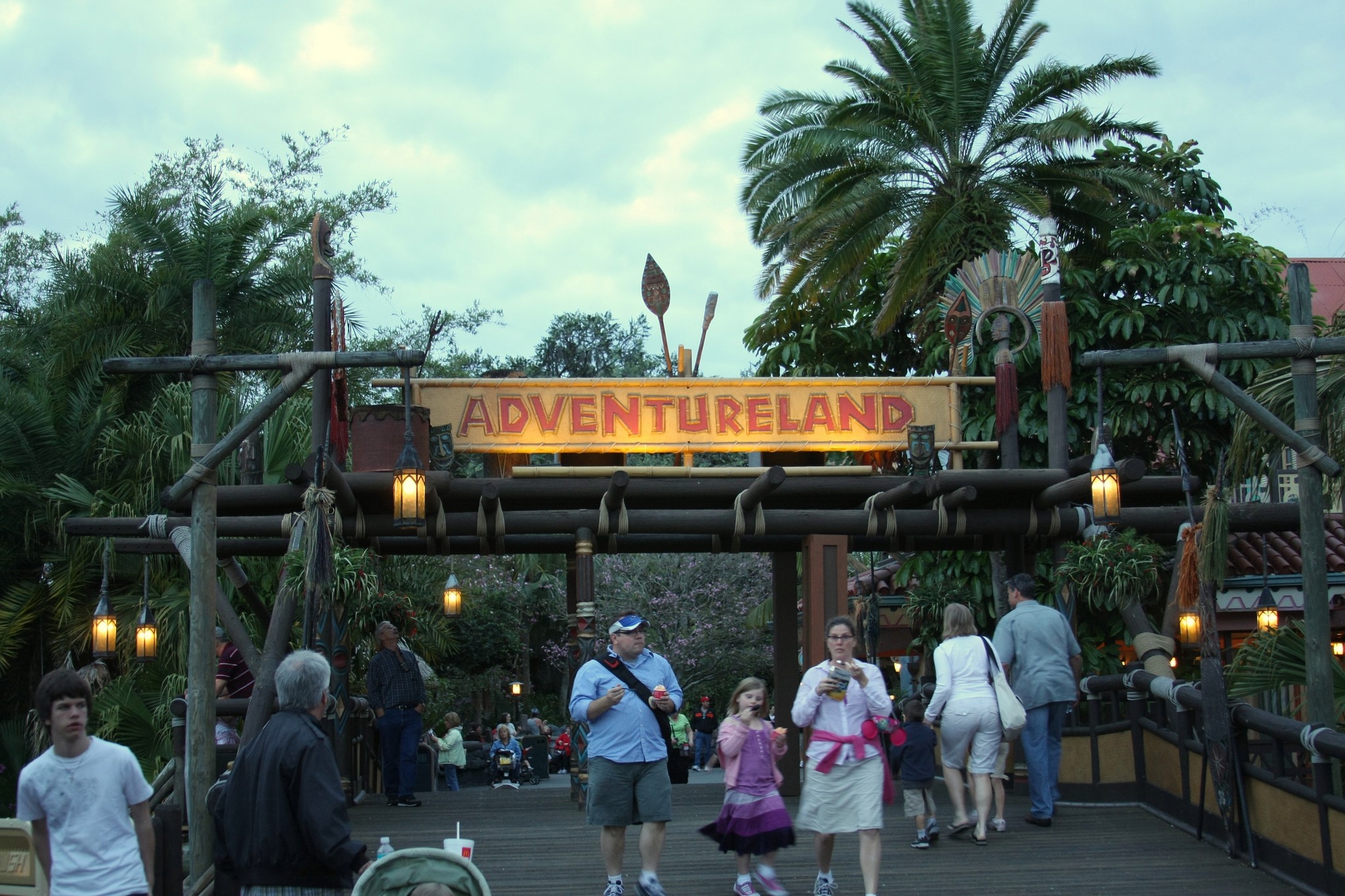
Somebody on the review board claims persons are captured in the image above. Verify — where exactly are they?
[889,603,1011,847]
[183,626,255,746]
[16,669,155,896]
[569,610,683,896]
[762,704,776,729]
[704,708,734,772]
[690,696,718,772]
[991,574,1081,827]
[430,707,571,791]
[366,620,425,807]
[695,677,797,896]
[214,649,374,896]
[668,708,693,784]
[791,615,893,896]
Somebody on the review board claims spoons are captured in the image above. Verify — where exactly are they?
[737,704,760,718]
[612,687,637,691]
[837,659,858,666]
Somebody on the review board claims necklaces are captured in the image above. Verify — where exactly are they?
[55,739,91,781]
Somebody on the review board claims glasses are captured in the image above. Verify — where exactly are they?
[380,625,394,635]
[614,629,648,637]
[828,635,854,641]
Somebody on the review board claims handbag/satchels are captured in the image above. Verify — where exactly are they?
[979,637,1027,731]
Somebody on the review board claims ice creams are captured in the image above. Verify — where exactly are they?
[653,684,667,700]
[770,726,787,741]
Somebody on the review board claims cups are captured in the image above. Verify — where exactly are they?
[560,741,562,745]
[443,838,475,861]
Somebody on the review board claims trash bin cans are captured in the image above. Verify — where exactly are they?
[514,735,550,780]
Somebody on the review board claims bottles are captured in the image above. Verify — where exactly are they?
[683,743,689,756]
[377,837,394,860]
[556,750,561,754]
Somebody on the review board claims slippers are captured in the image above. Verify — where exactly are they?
[946,822,972,836]
[970,833,988,845]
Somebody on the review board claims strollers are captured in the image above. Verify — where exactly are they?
[519,763,541,785]
[348,848,494,896]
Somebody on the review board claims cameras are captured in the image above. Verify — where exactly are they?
[428,729,434,736]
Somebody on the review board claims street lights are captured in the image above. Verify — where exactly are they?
[102,277,430,896]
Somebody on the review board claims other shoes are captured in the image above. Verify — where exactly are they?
[989,819,1006,831]
[558,769,563,774]
[528,767,534,771]
[562,770,568,774]
[968,813,978,823]
[692,765,701,771]
[1025,814,1052,828]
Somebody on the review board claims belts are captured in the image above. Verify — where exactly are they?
[393,704,412,710]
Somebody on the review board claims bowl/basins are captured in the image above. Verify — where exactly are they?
[653,689,667,700]
[770,729,781,741]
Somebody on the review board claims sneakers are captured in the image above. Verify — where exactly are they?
[926,822,940,841]
[733,879,760,896]
[911,835,929,847]
[603,879,624,896]
[387,796,399,805]
[753,866,789,895]
[812,872,838,896]
[634,875,668,896]
[397,797,422,806]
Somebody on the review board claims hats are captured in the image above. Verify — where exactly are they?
[532,708,538,713]
[214,627,227,637]
[701,697,710,701]
[608,615,651,635]
